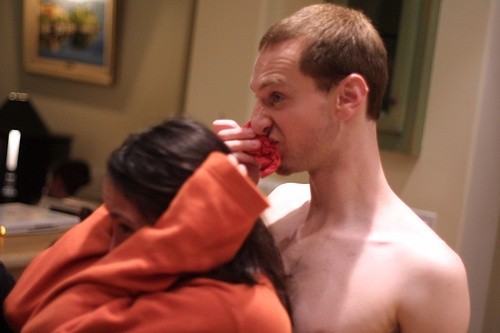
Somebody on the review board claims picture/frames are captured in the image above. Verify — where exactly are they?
[324,0,442,158]
[20,0,121,86]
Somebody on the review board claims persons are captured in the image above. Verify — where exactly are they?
[3,117,294,333]
[212,2,471,333]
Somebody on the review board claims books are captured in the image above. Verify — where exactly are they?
[0,202,81,236]
